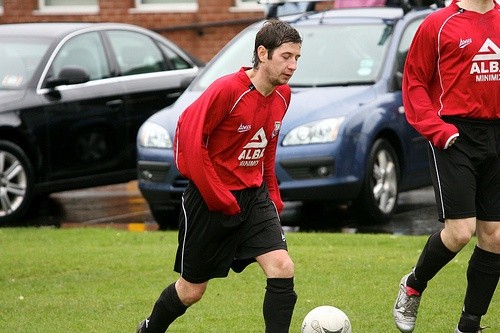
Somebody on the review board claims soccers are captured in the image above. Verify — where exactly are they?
[300,305,351,333]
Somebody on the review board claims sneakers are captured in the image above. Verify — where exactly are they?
[456,305,484,333]
[393,272,423,332]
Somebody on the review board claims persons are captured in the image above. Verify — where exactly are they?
[391,0,499,333]
[136,16,302,332]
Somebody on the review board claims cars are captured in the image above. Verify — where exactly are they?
[137,1,449,228]
[1,20,209,233]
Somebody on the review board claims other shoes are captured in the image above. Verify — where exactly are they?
[136,315,150,332]
